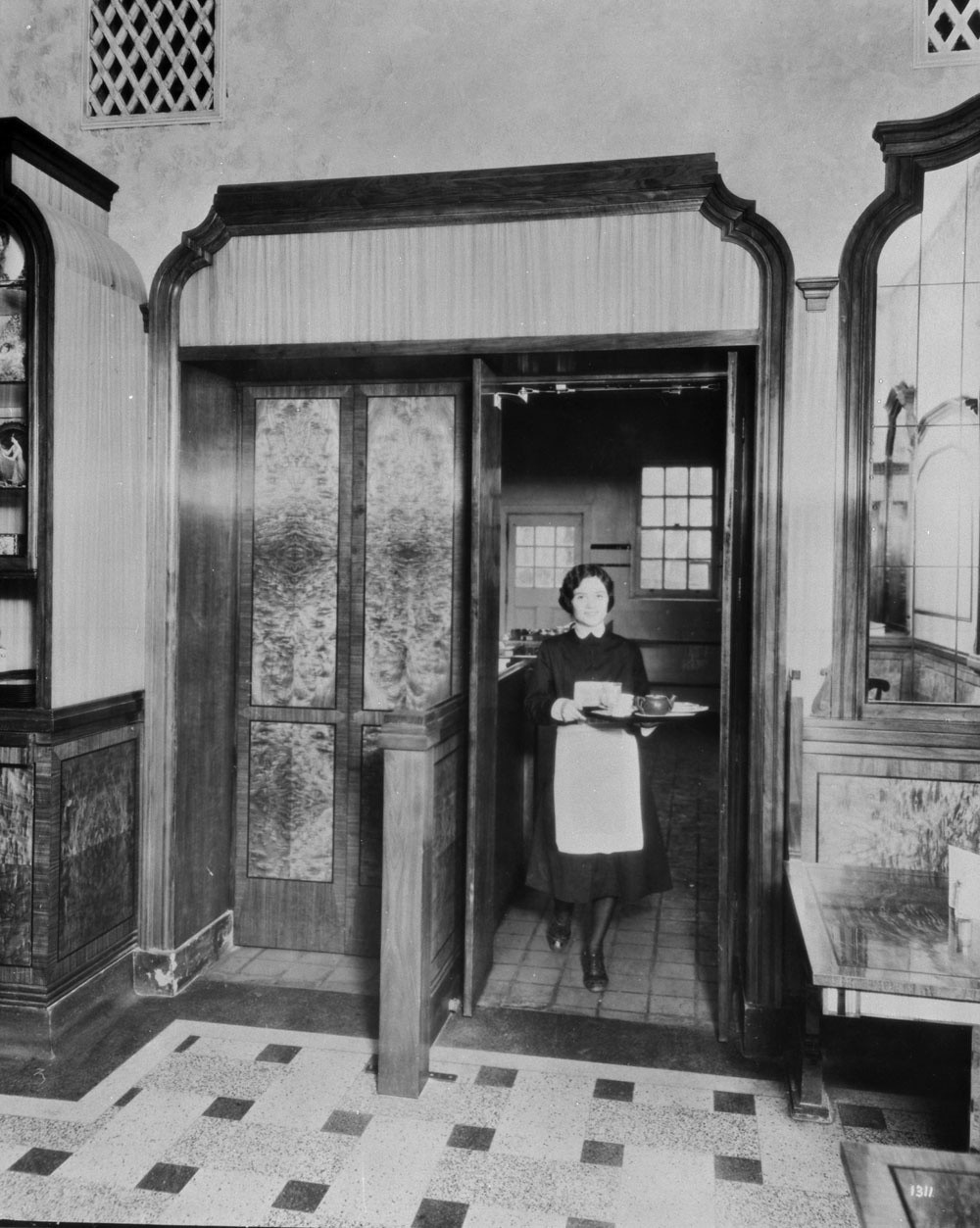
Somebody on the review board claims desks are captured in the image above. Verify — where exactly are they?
[835,1139,980,1228]
[774,854,980,1151]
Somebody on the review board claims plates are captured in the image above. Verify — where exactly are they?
[0,421,27,486]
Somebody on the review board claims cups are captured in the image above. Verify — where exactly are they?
[599,681,622,710]
[510,625,570,642]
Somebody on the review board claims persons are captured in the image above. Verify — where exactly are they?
[519,564,658,993]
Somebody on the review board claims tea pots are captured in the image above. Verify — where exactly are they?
[637,694,676,716]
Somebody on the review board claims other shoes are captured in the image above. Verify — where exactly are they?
[582,946,608,991]
[547,922,572,952]
